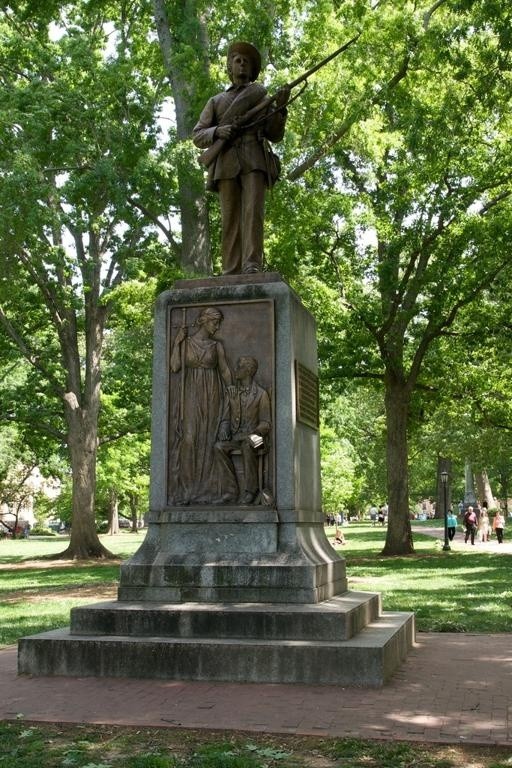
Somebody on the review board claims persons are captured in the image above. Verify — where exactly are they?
[212,356,272,504]
[191,42,293,279]
[168,307,236,505]
[328,497,505,546]
